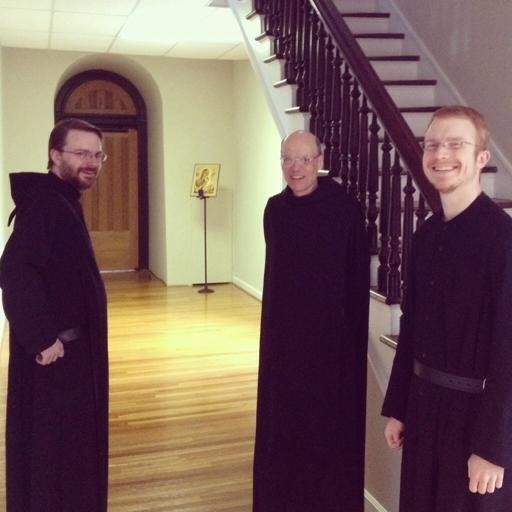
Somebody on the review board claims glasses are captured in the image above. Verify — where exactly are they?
[57,149,107,162]
[419,138,485,152]
[279,153,321,166]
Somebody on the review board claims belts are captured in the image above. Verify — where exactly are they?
[58,324,85,342]
[412,360,488,395]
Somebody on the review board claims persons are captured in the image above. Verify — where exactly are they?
[191,163,220,198]
[252,130,371,512]
[0,117,109,512]
[380,105,512,512]
